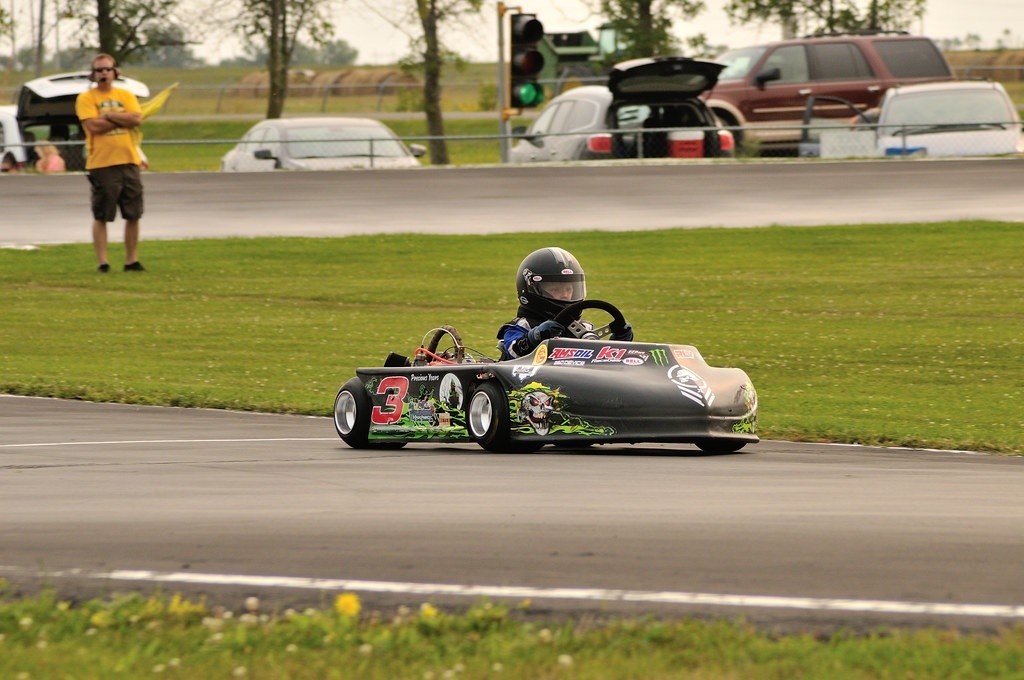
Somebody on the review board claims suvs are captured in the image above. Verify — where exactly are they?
[696,30,955,146]
[0,69,151,172]
[512,55,737,159]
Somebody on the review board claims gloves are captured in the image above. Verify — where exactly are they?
[529,320,566,347]
[611,325,635,342]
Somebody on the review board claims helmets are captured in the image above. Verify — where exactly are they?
[516,247,586,323]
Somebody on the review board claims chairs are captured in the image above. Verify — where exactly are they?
[24,131,34,153]
[49,136,65,151]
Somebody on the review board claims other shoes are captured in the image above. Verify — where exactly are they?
[98,263,110,272]
[124,261,147,272]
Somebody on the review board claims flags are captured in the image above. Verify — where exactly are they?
[141,87,174,117]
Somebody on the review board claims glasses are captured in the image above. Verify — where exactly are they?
[92,66,114,73]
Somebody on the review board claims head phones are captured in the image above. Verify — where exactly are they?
[89,55,120,82]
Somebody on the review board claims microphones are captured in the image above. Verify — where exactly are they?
[98,77,106,82]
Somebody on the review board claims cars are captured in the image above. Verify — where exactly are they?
[221,118,428,169]
[798,79,1024,160]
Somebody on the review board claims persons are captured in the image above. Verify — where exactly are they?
[497,247,634,360]
[34,140,65,171]
[76,54,145,272]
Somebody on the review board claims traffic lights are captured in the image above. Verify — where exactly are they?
[508,15,543,109]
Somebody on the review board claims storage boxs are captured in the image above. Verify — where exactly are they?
[667,130,703,158]
